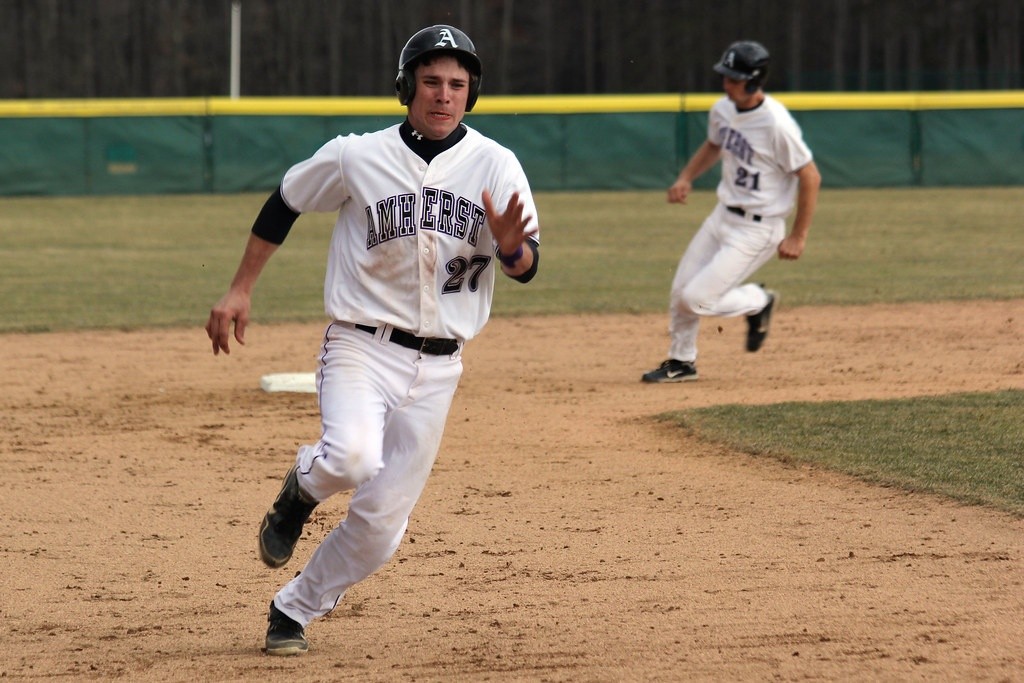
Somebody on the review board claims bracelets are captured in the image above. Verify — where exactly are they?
[498,244,523,268]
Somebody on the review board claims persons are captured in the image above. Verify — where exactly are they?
[638,39,824,385]
[203,20,544,660]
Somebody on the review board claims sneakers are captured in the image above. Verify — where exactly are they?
[266,600,308,655]
[642,359,699,382]
[747,284,779,348]
[259,465,320,568]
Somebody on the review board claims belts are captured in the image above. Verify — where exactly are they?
[355,324,458,355]
[728,207,761,221]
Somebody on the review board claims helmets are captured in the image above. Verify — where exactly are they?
[395,25,482,112]
[712,41,771,93]
